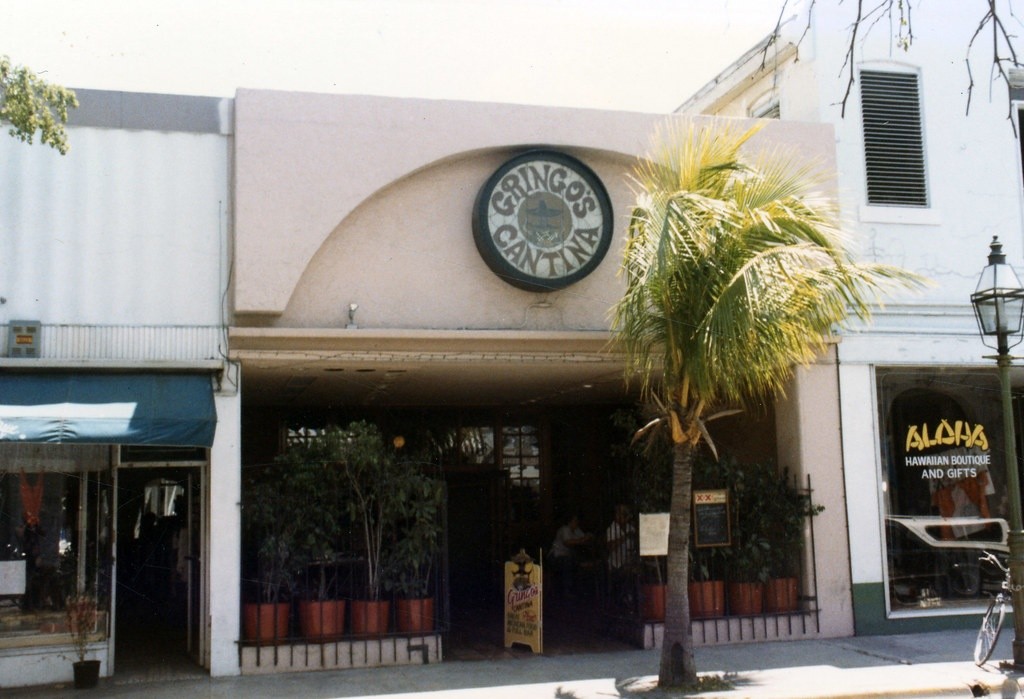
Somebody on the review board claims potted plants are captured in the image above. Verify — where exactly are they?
[240,420,446,645]
[622,446,826,622]
[63,585,110,690]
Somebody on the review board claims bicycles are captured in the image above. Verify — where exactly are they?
[974,549,1010,668]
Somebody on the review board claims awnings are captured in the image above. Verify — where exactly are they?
[0,370,217,448]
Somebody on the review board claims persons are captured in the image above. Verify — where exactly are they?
[603,502,637,569]
[546,513,596,600]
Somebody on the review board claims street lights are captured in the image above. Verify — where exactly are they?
[971,233,1023,699]
